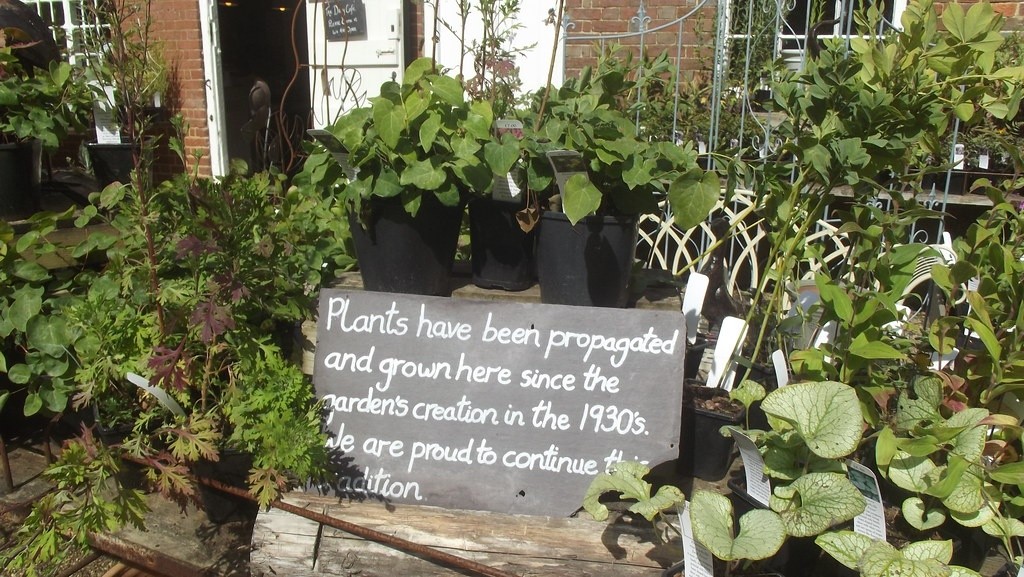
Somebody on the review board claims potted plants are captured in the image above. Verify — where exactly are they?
[82,36,172,202]
[0,113,332,577]
[581,379,868,577]
[873,379,1024,577]
[463,85,550,292]
[302,51,520,297]
[519,94,720,308]
[0,46,95,219]
[638,86,1024,195]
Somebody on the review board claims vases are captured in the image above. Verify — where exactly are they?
[684,336,708,382]
[682,383,746,481]
